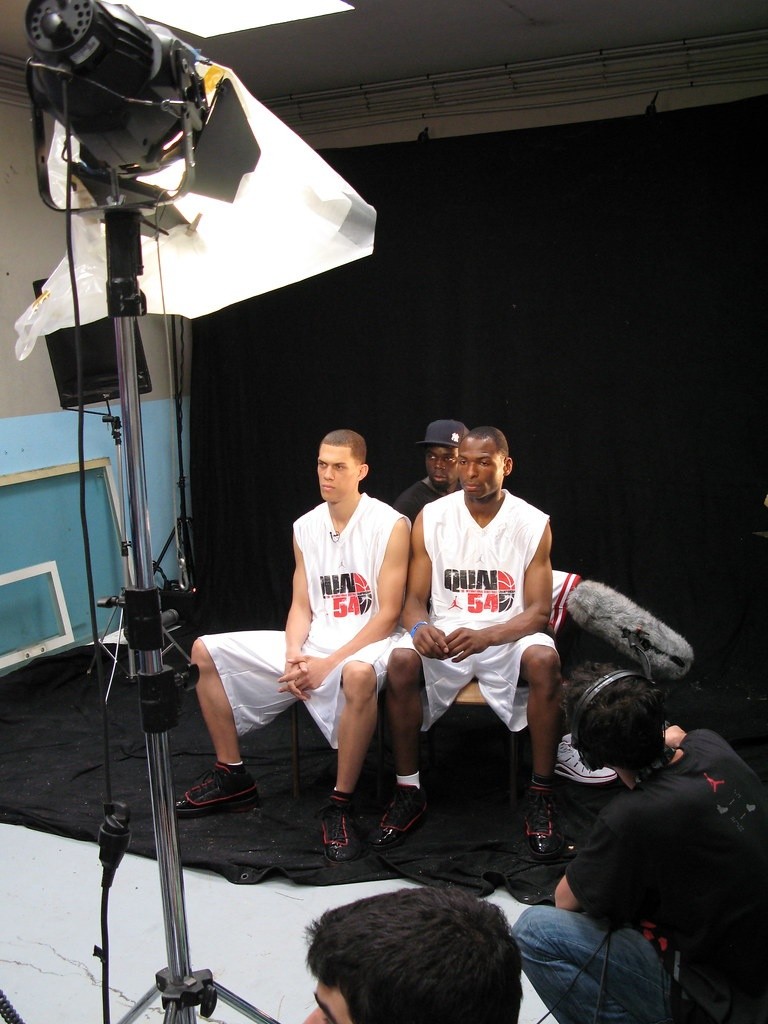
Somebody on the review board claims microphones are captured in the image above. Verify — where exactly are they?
[568,582,693,680]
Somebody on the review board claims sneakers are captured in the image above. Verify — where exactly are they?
[366,781,430,849]
[176,764,259,818]
[554,733,619,785]
[520,787,565,858]
[315,798,361,865]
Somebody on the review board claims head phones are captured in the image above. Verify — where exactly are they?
[571,669,665,772]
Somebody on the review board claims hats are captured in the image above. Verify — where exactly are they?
[415,419,470,448]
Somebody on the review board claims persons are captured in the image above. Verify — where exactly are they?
[512,660,768,1024]
[371,426,563,858]
[175,428,412,863]
[306,887,523,1024]
[393,421,616,783]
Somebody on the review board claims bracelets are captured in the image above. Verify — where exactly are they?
[410,621,428,638]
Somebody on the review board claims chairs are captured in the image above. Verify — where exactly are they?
[291,689,385,808]
[456,681,522,810]
